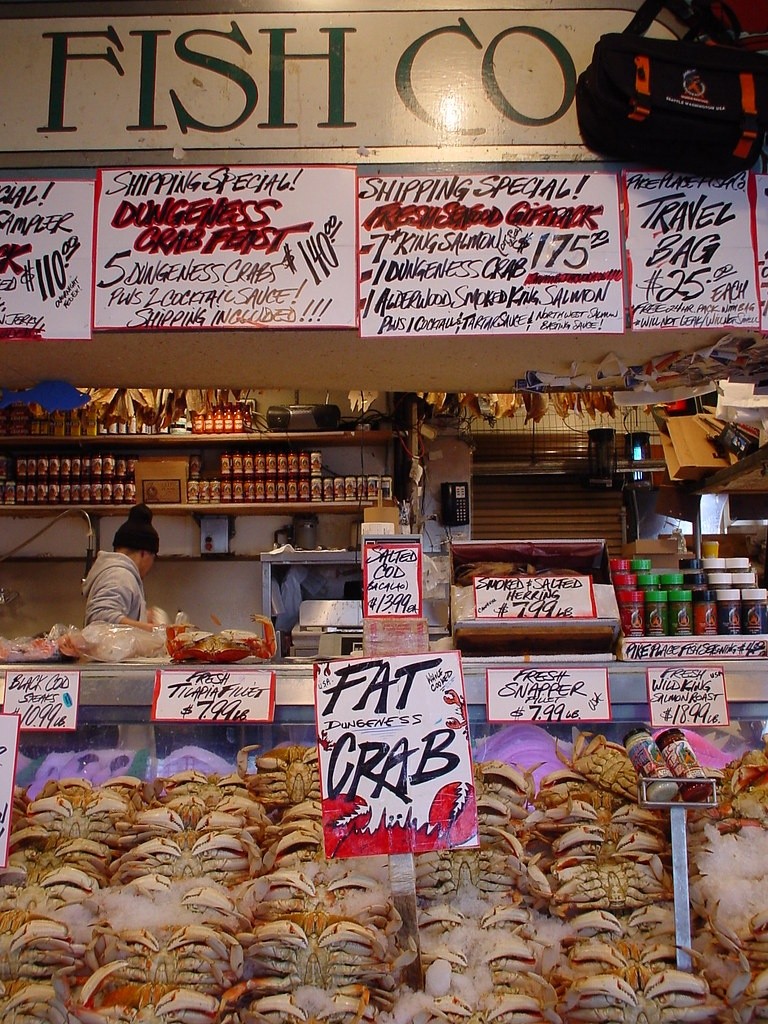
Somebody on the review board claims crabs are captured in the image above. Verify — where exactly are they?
[1,726,768,1024]
[166,612,278,664]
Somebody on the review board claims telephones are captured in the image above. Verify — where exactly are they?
[440,481,469,528]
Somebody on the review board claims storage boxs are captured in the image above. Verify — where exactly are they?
[659,415,738,481]
[450,539,620,656]
[134,456,188,505]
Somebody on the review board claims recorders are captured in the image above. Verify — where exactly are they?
[266,393,341,432]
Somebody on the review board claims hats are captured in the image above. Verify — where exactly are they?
[113,504,160,554]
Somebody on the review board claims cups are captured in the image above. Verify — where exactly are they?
[702,541,718,558]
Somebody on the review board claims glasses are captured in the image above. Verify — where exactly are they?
[154,553,159,562]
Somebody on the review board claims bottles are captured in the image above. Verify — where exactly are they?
[99,401,252,433]
[611,559,768,637]
[0,452,136,504]
[622,728,679,802]
[655,729,712,803]
[187,450,393,504]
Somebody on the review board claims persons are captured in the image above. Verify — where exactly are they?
[80,502,164,775]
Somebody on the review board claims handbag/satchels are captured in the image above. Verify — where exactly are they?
[575,0,768,179]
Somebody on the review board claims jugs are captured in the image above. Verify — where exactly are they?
[274,512,319,550]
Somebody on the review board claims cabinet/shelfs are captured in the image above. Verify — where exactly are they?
[0,429,396,515]
